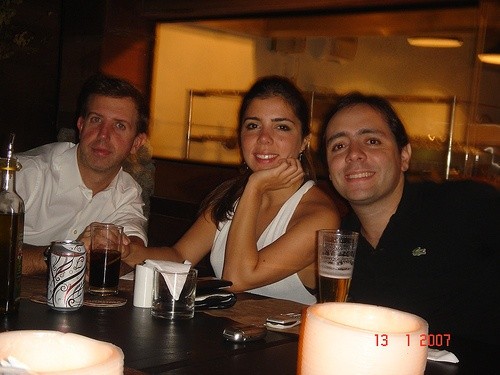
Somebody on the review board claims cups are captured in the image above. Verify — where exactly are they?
[1,329,125,375]
[315,229,360,304]
[297,300,430,375]
[85,222,125,298]
[150,267,200,321]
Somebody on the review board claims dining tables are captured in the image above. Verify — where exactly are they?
[0,272,499,375]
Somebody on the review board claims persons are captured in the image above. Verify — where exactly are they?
[118,75,340,306]
[0,73,148,277]
[318,95,500,375]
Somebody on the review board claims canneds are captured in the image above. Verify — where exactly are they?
[45,239,87,314]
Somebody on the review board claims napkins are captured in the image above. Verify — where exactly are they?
[145,259,192,300]
[427,348,459,364]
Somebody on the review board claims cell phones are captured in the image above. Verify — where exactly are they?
[222,326,267,342]
[266,312,303,329]
[184,290,237,312]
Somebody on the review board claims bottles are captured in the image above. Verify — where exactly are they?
[0,132,26,334]
